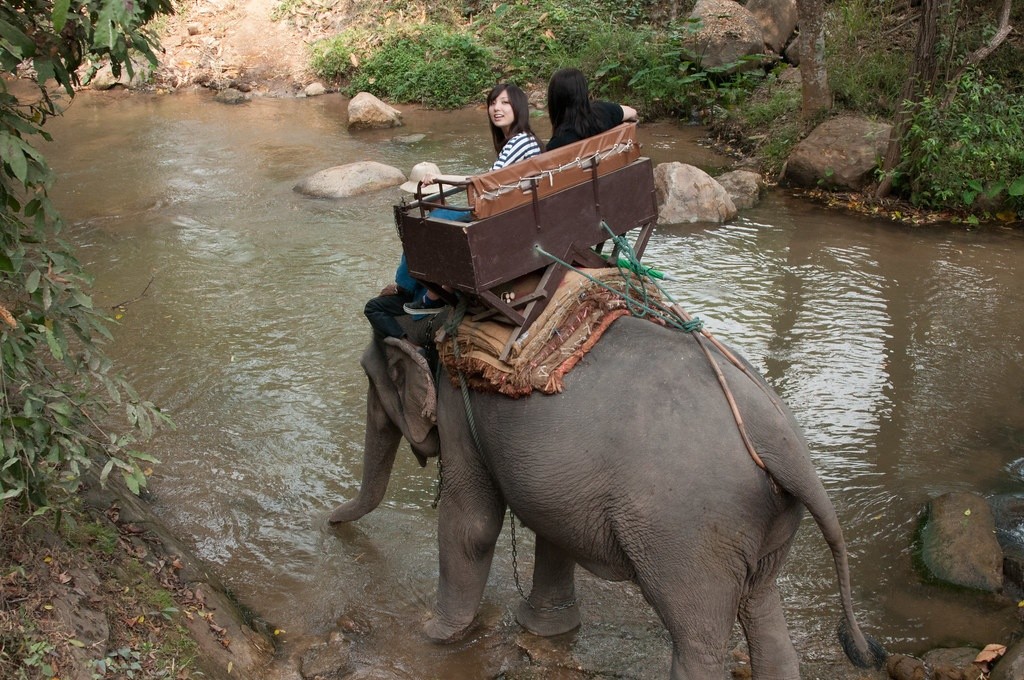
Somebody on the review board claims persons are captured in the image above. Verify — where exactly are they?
[545,68,636,153]
[364,161,470,358]
[403,84,545,314]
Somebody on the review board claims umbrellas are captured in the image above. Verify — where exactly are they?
[589,247,679,282]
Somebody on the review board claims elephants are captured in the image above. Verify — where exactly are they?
[327,265,889,680]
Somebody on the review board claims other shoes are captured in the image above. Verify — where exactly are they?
[403,292,459,314]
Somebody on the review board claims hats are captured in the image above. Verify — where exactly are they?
[400,161,473,194]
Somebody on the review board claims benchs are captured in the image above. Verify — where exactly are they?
[417,118,640,221]
